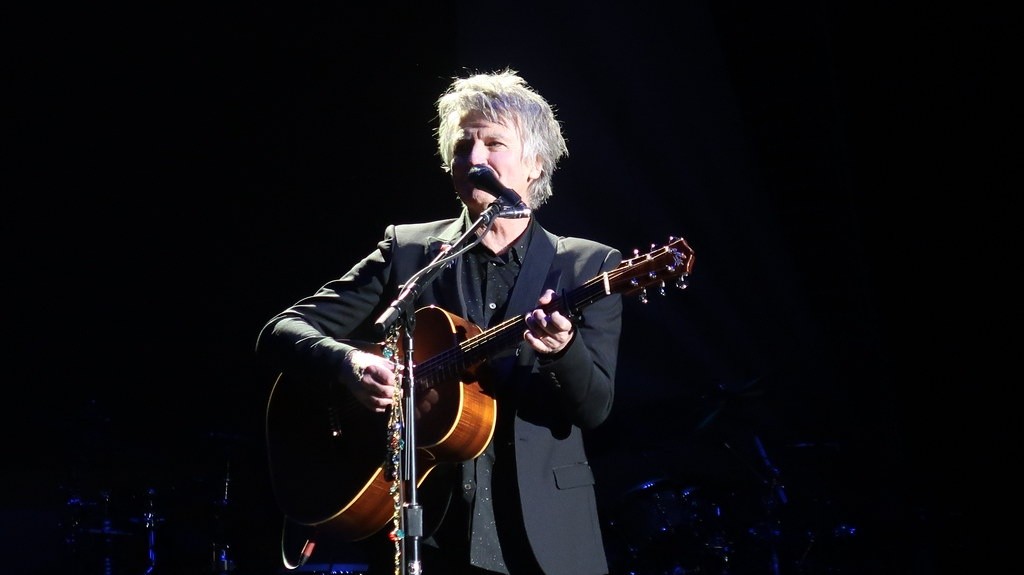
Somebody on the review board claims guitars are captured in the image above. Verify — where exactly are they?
[263,233,696,546]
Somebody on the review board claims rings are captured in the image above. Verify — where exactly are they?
[540,334,548,339]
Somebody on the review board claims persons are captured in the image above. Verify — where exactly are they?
[255,74,622,575]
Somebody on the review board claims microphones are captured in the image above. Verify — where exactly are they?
[467,165,530,216]
